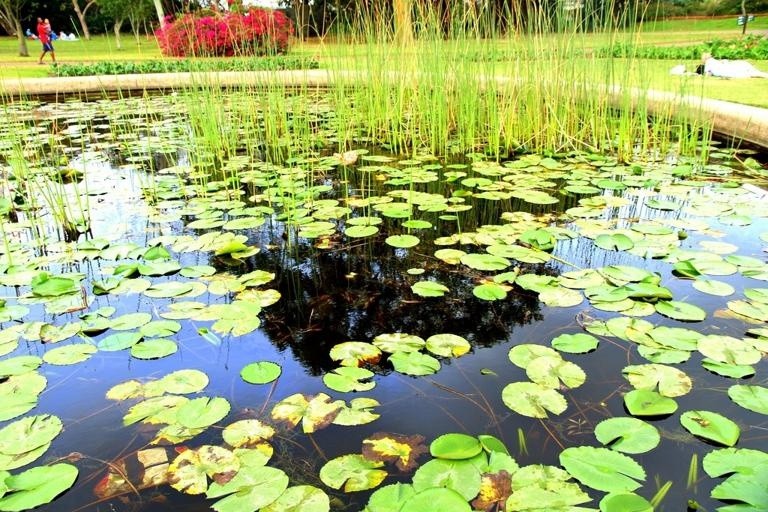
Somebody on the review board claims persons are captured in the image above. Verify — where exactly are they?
[697,52,768,78]
[26,17,76,65]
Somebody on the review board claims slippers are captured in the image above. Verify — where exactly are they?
[39,62,46,64]
